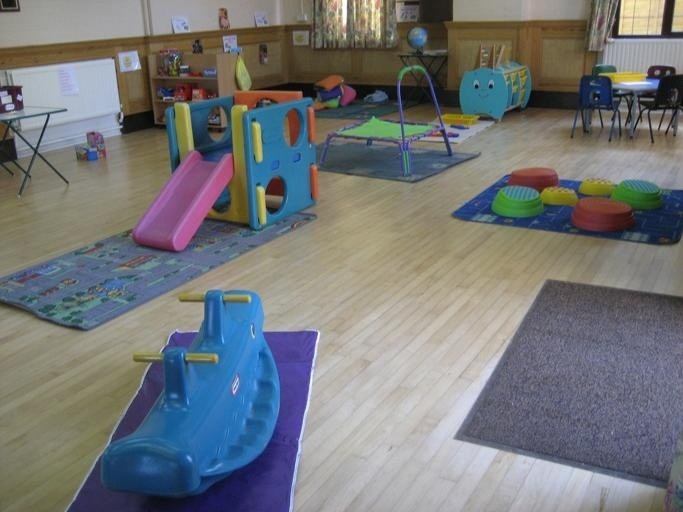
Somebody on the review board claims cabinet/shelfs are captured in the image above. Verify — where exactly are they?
[147,51,247,130]
[458,60,531,123]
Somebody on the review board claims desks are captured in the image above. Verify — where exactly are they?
[397,51,447,106]
[607,79,659,141]
[0,106,70,197]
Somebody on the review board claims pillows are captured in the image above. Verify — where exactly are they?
[311,75,356,110]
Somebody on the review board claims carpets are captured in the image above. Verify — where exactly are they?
[0,212,316,331]
[64,331,322,510]
[311,96,418,121]
[454,279,682,489]
[453,171,682,246]
[415,117,494,145]
[314,140,480,183]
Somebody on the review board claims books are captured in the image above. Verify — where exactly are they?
[598,72,646,84]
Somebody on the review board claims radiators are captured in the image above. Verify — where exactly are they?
[4,56,123,133]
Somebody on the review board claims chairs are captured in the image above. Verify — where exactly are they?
[624,65,676,128]
[569,75,634,142]
[591,64,632,129]
[631,74,682,143]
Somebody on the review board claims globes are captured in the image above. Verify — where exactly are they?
[407,26,428,55]
